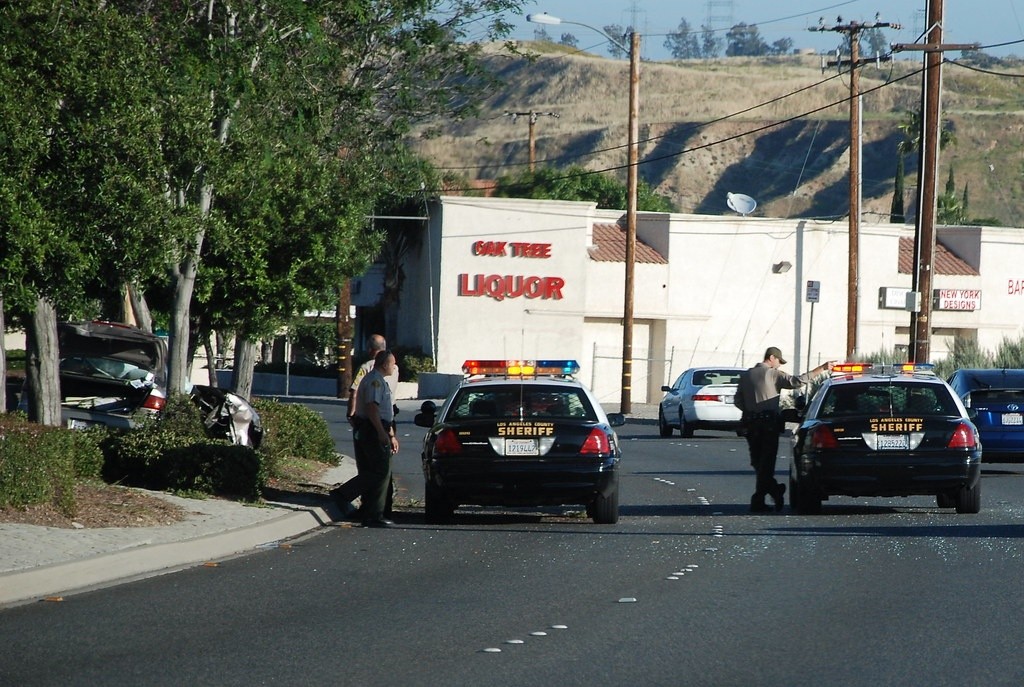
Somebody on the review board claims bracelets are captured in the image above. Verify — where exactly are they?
[391,436,395,437]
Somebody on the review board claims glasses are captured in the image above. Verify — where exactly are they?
[386,349,391,355]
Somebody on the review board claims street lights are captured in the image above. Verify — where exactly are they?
[527,13,640,414]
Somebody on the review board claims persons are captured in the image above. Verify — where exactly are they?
[328,334,400,528]
[734,347,838,512]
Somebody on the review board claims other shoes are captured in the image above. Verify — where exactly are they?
[332,490,350,517]
[751,495,774,512]
[364,515,398,528]
[767,482,786,512]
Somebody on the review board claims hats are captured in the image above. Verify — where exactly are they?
[764,347,789,366]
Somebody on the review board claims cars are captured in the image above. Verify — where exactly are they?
[16,320,170,433]
[790,363,983,515]
[659,366,748,439]
[945,368,1024,464]
[414,359,621,525]
